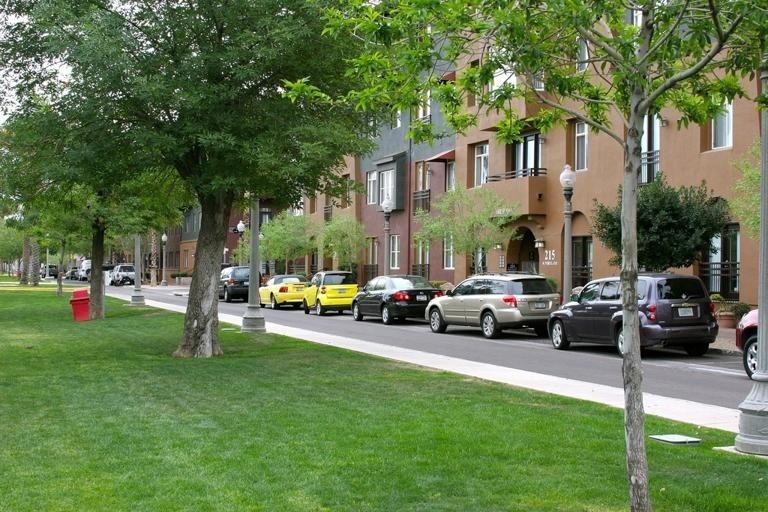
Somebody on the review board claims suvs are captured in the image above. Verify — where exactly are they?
[424,270,563,339]
[546,272,718,358]
[219,266,263,303]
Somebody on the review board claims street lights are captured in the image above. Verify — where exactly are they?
[237,220,245,266]
[381,192,394,276]
[258,232,265,276]
[161,232,168,286]
[559,164,577,307]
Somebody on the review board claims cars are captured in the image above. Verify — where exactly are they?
[303,271,359,316]
[351,274,446,325]
[736,308,758,380]
[259,275,311,309]
[39,259,135,286]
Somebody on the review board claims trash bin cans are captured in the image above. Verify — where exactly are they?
[69,289,90,321]
